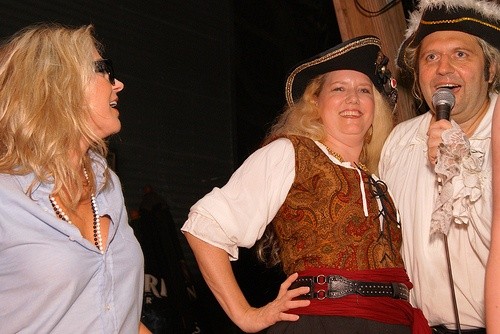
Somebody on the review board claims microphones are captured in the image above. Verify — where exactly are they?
[432,88,456,122]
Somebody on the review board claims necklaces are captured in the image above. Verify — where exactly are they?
[48,167,105,255]
[317,139,345,163]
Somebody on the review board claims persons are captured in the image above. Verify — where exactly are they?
[179,31,434,334]
[376,0,500,334]
[0,24,154,334]
[129,189,202,334]
